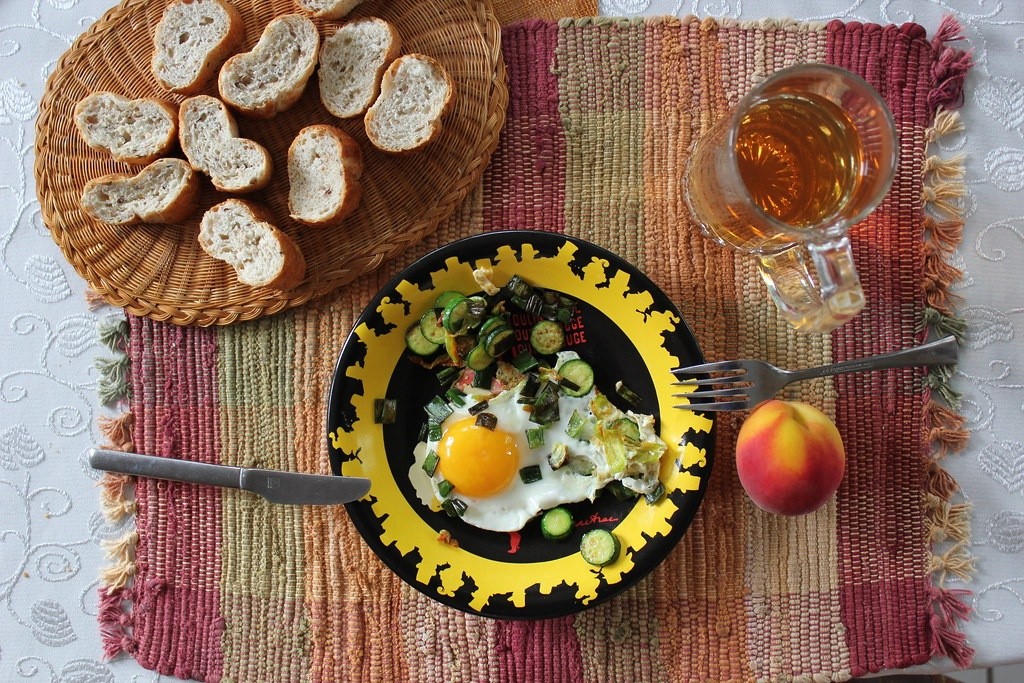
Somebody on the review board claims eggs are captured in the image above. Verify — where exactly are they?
[407,351,667,532]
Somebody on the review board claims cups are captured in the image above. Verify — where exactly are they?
[682,60,899,334]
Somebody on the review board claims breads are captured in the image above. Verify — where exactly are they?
[72,0,458,289]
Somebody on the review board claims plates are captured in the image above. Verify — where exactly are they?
[324,220,717,622]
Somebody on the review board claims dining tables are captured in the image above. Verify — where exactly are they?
[0,0,1024,682]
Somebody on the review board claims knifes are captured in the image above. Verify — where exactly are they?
[89,446,373,507]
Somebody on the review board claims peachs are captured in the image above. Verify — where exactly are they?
[735,400,846,517]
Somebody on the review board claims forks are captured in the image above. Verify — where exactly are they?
[670,333,961,412]
